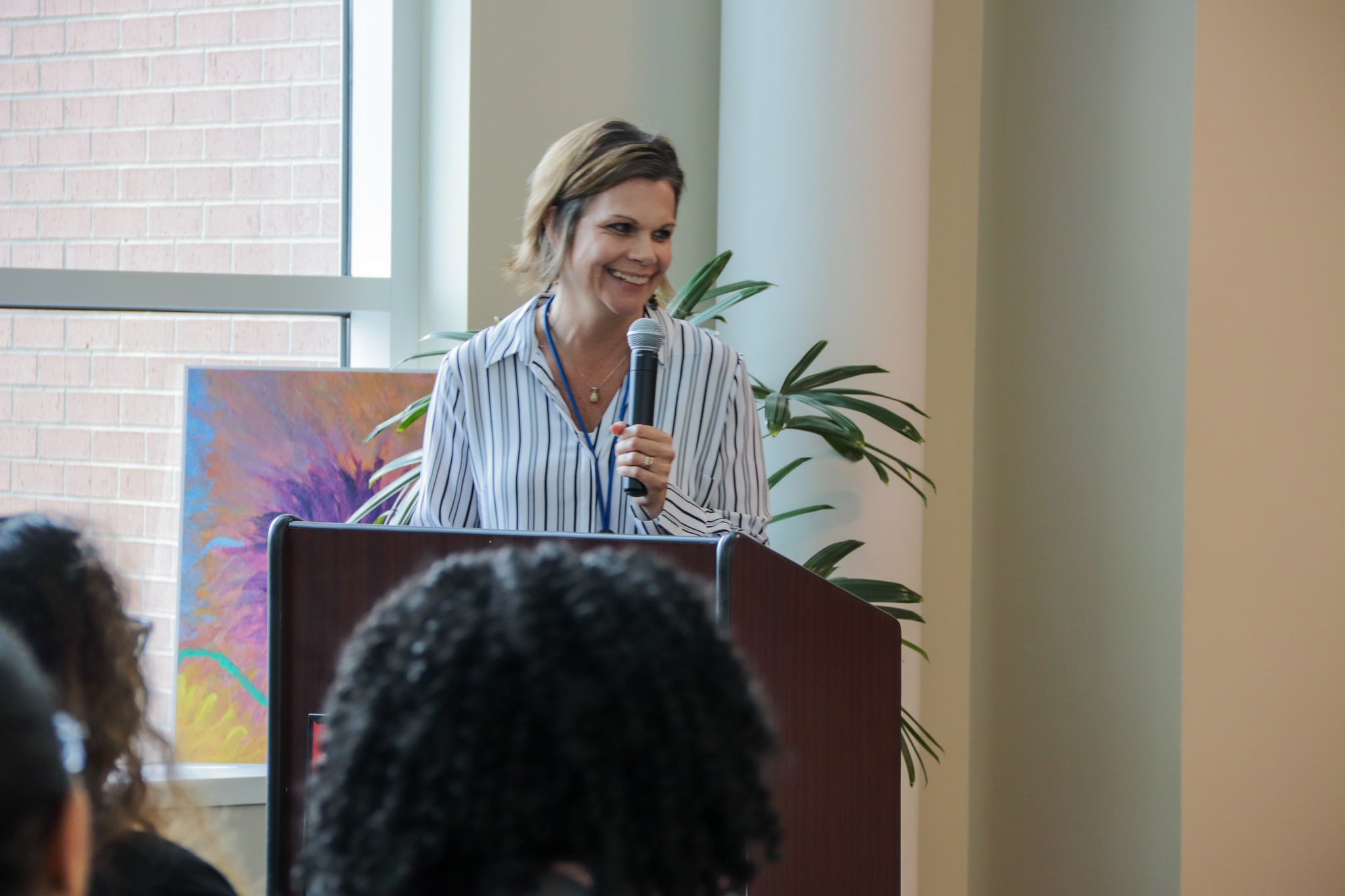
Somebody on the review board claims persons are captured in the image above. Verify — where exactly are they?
[405,115,775,545]
[0,512,249,896]
[291,546,787,896]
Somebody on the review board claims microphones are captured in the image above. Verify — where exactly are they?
[623,318,665,498]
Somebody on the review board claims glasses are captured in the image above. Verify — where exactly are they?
[47,711,86,776]
[109,615,152,657]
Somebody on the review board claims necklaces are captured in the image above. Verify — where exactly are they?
[547,306,631,403]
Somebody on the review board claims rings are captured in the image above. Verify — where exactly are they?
[642,456,654,469]
